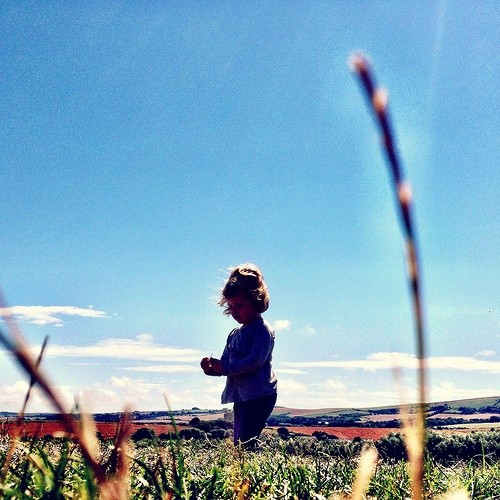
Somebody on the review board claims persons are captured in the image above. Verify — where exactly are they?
[199,264,281,453]
